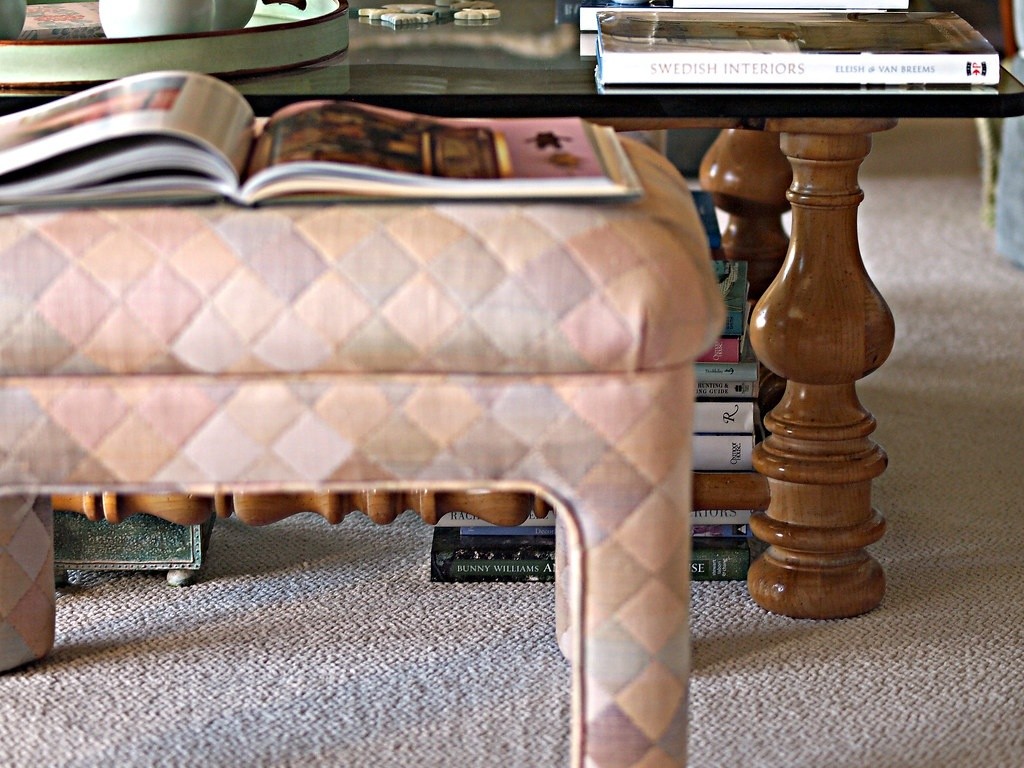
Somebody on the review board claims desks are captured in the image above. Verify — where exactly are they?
[0,0,1024,621]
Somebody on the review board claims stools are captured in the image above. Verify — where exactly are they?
[0,134,724,768]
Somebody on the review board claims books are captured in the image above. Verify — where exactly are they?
[431,191,765,582]
[577,0,999,85]
[0,69,646,208]
